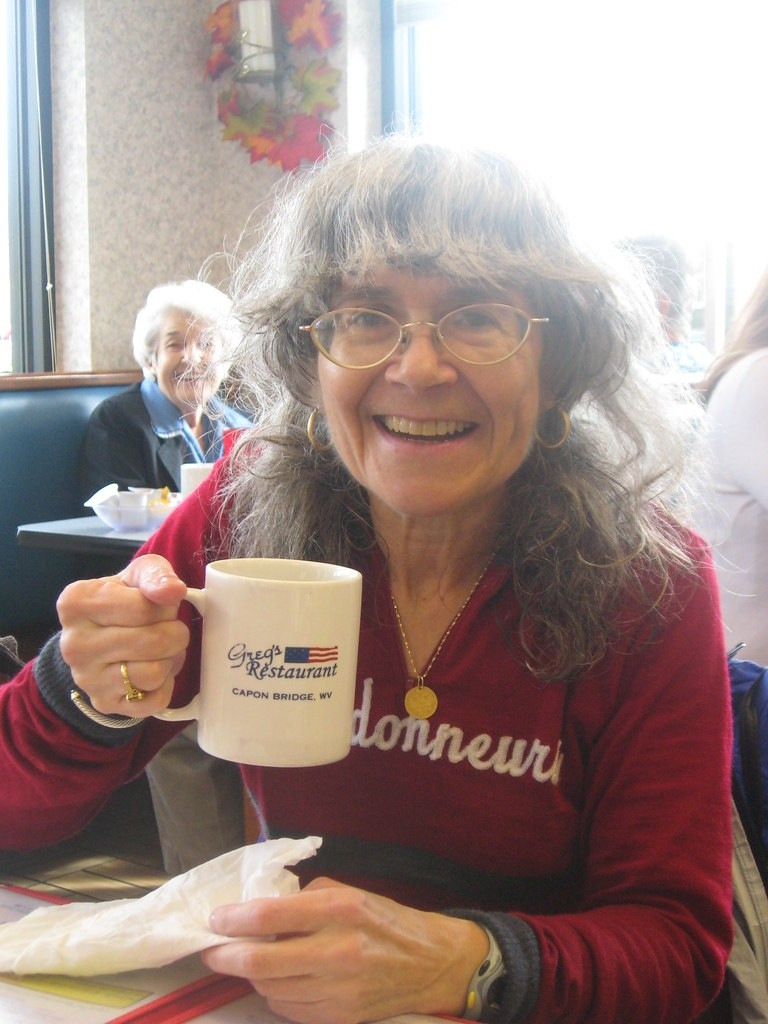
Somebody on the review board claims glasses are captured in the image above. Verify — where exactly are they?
[296,302,551,372]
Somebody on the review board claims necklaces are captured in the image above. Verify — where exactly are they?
[390,547,501,719]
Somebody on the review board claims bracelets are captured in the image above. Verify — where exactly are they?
[69,689,146,728]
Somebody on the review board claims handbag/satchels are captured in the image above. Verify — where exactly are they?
[726,642,768,897]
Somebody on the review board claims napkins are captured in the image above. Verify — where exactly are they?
[0,834,324,978]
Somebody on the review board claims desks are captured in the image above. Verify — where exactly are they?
[0,884,486,1024]
[17,516,150,559]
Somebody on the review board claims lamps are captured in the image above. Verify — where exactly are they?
[232,0,282,85]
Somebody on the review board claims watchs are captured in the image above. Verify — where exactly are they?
[464,923,506,1022]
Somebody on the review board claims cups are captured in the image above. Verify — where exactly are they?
[181,463,213,504]
[151,557,362,766]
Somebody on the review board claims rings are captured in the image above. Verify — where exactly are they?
[121,661,145,702]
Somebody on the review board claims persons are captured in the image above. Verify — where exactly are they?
[677,265,768,667]
[0,134,734,1024]
[76,279,255,517]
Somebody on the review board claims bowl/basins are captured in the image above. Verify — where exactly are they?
[91,493,181,531]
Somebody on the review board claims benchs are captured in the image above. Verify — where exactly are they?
[0,366,267,664]
